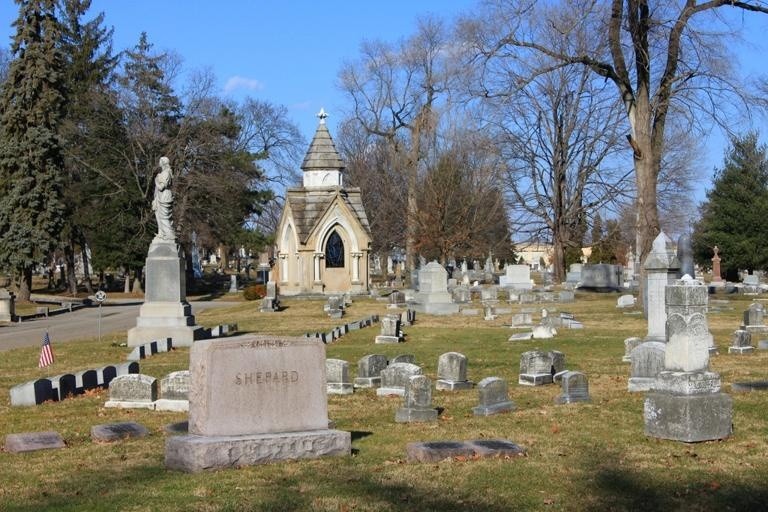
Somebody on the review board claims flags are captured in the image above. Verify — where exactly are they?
[38,330,56,369]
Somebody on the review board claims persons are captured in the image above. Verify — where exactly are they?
[151,156,179,240]
